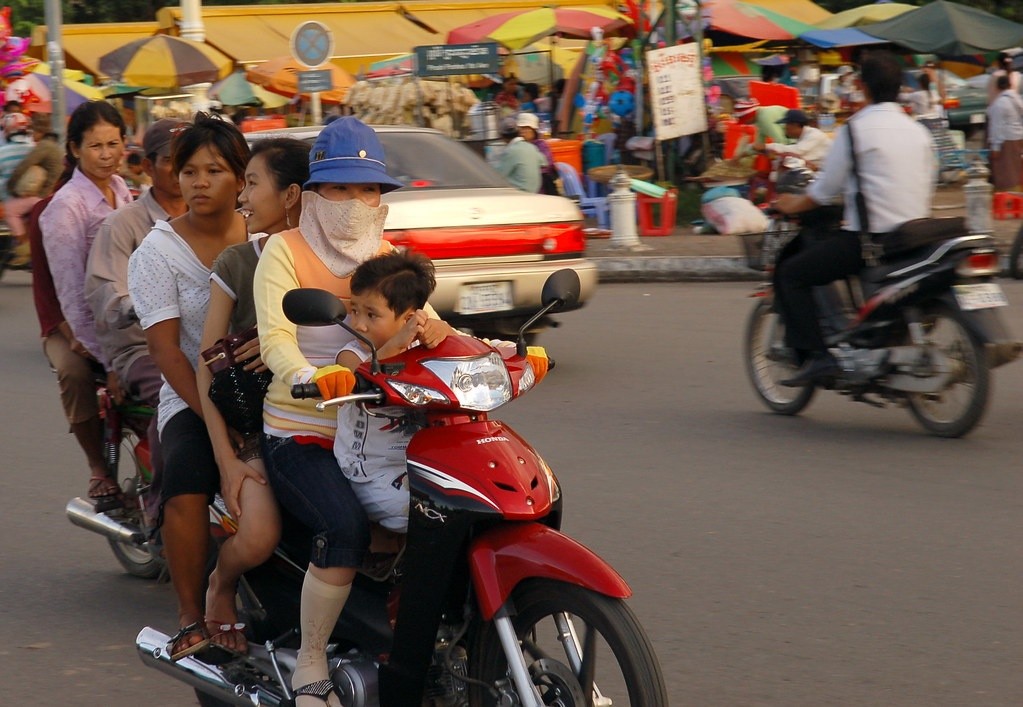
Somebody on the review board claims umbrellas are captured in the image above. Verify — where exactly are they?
[445,0,1023,133]
[0,70,103,119]
[96,32,359,109]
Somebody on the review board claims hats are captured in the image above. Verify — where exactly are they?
[836,65,854,76]
[27,116,56,133]
[515,113,540,129]
[302,116,405,195]
[143,118,187,157]
[775,109,810,124]
[498,117,518,134]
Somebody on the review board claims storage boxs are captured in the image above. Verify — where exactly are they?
[941,148,992,167]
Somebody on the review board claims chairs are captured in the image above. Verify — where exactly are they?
[552,161,610,228]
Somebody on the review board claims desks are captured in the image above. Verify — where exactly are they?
[587,163,655,199]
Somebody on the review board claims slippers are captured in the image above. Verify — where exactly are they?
[289,669,335,707]
[87,472,118,500]
[204,614,249,656]
[166,621,210,661]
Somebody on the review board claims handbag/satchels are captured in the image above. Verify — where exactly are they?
[200,320,273,435]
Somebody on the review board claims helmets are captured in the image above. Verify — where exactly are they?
[0,113,30,138]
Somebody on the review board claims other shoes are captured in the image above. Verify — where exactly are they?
[775,355,840,387]
[880,215,968,264]
[7,244,33,266]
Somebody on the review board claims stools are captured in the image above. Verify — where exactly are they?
[636,185,681,237]
[992,191,1023,220]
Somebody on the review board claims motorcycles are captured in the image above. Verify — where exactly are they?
[63,406,164,585]
[134,266,669,707]
[744,170,1023,438]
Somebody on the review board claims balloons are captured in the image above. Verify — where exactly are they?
[0,6,53,114]
[569,26,638,143]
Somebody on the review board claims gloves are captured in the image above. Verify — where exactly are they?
[526,345,549,387]
[308,363,356,409]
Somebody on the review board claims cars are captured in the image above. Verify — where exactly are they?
[242,123,597,336]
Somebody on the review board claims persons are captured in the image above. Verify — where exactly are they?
[488,117,549,194]
[986,51,1023,184]
[331,245,484,538]
[772,54,794,87]
[984,74,1023,210]
[769,51,942,387]
[516,112,562,200]
[495,72,522,112]
[516,83,539,114]
[752,108,850,228]
[27,97,524,707]
[897,73,942,132]
[831,65,868,110]
[0,99,66,267]
[732,98,809,166]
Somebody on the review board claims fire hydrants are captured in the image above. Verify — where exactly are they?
[605,168,639,251]
